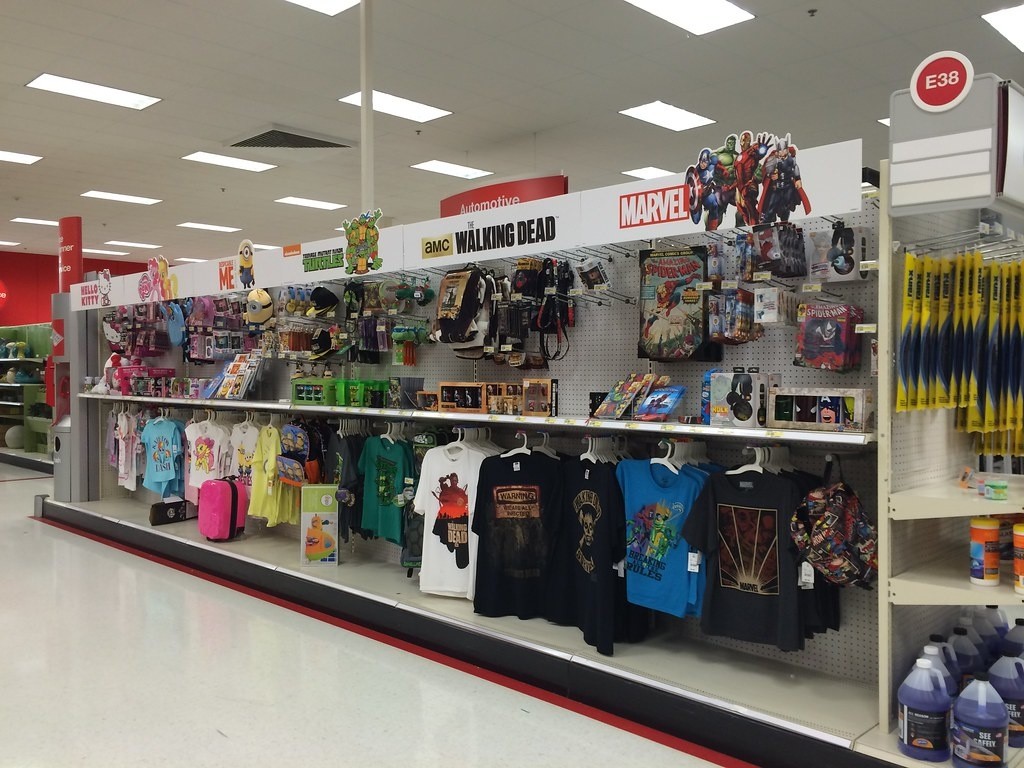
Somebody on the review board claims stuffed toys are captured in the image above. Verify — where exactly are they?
[242,289,276,337]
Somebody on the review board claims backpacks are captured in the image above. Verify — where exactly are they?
[790,453,880,591]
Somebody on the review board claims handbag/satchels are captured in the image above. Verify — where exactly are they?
[427,263,511,360]
[343,282,364,333]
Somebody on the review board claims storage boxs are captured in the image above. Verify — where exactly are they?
[301,484,339,566]
[810,226,871,283]
[291,379,389,407]
[710,373,874,432]
[416,376,558,416]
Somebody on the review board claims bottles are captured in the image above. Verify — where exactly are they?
[970,513,1024,595]
[897,605,1024,768]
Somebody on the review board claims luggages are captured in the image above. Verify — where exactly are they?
[197,475,248,542]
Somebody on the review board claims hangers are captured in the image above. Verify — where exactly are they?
[112,401,288,428]
[336,419,372,439]
[444,424,500,450]
[725,438,798,474]
[379,421,415,444]
[580,433,635,465]
[500,431,560,460]
[650,441,711,475]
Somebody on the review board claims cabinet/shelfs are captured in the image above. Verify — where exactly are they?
[0,322,54,475]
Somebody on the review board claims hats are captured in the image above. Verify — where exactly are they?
[308,330,331,360]
[306,287,339,318]
